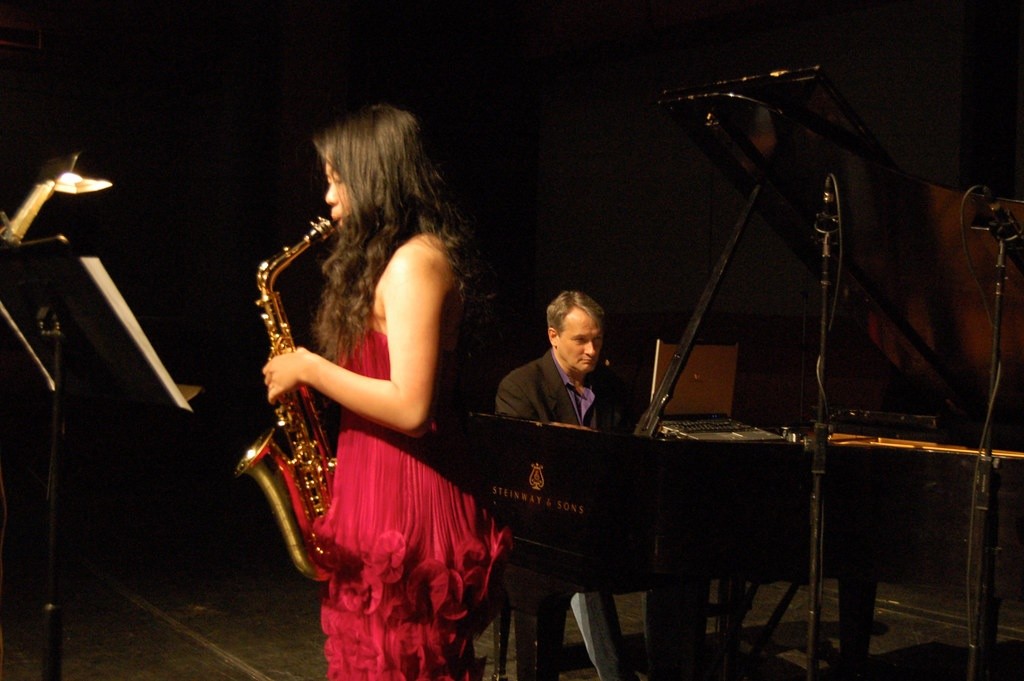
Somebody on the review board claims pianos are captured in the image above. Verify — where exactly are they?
[441,61,1024,681]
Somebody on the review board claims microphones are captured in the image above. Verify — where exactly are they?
[984,187,1024,276]
[813,175,834,245]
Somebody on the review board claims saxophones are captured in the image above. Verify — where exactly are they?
[233,215,338,582]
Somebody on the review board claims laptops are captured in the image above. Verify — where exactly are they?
[650,340,785,441]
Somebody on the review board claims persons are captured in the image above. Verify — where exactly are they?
[496,291,689,681]
[262,104,511,681]
